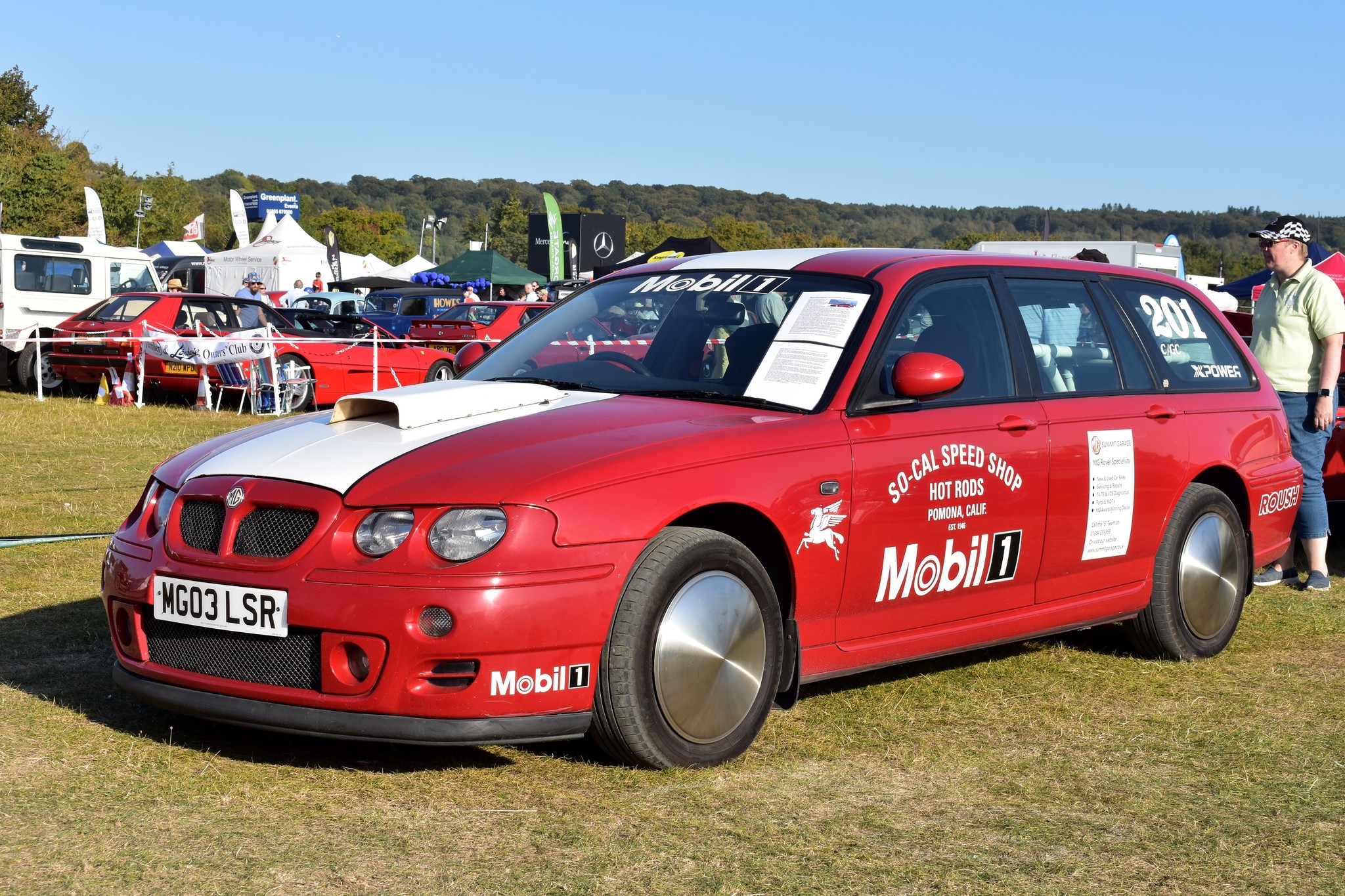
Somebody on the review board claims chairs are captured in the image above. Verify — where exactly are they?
[175,310,191,330]
[70,268,88,293]
[194,312,215,327]
[215,362,258,415]
[275,325,286,328]
[723,323,779,386]
[44,275,74,293]
[32,260,45,290]
[912,323,980,398]
[314,328,329,334]
[252,344,318,416]
[16,272,36,290]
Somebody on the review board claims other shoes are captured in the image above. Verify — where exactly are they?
[1304,570,1331,592]
[1253,566,1301,586]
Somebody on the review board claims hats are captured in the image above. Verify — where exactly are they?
[1248,216,1311,246]
[645,299,655,305]
[243,278,249,283]
[539,289,548,295]
[355,289,363,294]
[248,272,263,284]
[1077,248,1110,263]
[634,302,643,307]
[167,279,188,290]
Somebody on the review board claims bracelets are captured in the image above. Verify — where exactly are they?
[1318,389,1333,397]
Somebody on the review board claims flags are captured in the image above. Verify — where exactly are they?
[183,214,204,241]
[230,190,250,249]
[317,224,341,282]
[544,192,564,282]
[1163,235,1186,282]
[1219,252,1223,278]
[568,237,577,287]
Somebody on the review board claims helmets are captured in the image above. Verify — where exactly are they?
[304,287,314,292]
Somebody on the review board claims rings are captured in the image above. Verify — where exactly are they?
[1324,421,1328,422]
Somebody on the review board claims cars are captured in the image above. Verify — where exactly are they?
[46,292,460,415]
[288,292,378,333]
[99,248,1305,774]
[407,302,715,385]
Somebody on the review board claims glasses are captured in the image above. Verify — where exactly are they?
[177,289,182,291]
[532,285,537,287]
[468,289,472,291]
[1258,237,1294,248]
[260,288,266,290]
[536,292,538,294]
[251,282,260,285]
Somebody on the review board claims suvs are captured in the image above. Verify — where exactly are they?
[341,288,464,343]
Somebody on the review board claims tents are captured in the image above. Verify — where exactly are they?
[414,250,547,313]
[138,213,437,297]
[1216,240,1345,315]
[579,236,727,313]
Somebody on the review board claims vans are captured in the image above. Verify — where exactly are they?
[0,232,169,396]
[129,256,208,295]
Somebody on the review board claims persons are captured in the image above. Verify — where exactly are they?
[1018,248,1110,347]
[279,272,323,329]
[233,272,276,328]
[1248,214,1345,590]
[455,282,552,308]
[355,289,366,309]
[702,289,789,378]
[635,298,660,320]
[168,279,188,293]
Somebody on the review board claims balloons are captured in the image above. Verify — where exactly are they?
[412,271,490,292]
